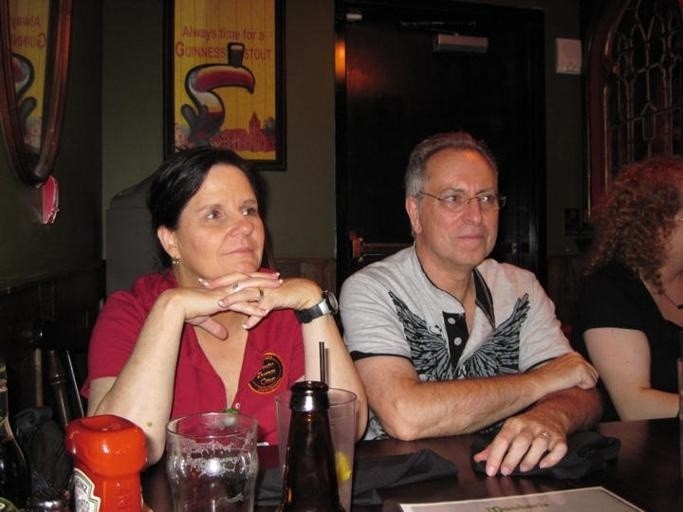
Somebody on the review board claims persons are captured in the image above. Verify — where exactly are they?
[380,433,570,512]
[560,151,683,423]
[76,144,374,479]
[336,130,609,477]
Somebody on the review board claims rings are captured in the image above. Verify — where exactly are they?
[232,282,239,293]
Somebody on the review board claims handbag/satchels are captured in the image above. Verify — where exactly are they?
[14,407,74,493]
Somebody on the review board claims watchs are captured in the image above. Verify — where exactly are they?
[293,290,340,324]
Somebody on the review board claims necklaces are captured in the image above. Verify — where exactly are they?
[654,282,683,311]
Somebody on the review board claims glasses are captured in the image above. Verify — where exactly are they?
[421,194,504,211]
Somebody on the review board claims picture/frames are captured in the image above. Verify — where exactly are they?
[0,0,74,189]
[161,0,289,175]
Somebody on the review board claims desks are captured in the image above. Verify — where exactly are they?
[138,416,683,510]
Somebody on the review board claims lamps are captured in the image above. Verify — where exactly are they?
[400,18,498,55]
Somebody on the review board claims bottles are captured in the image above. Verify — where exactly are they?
[281,380,344,511]
[0,364,31,509]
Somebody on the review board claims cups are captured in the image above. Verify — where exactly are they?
[272,387,356,512]
[163,411,260,512]
